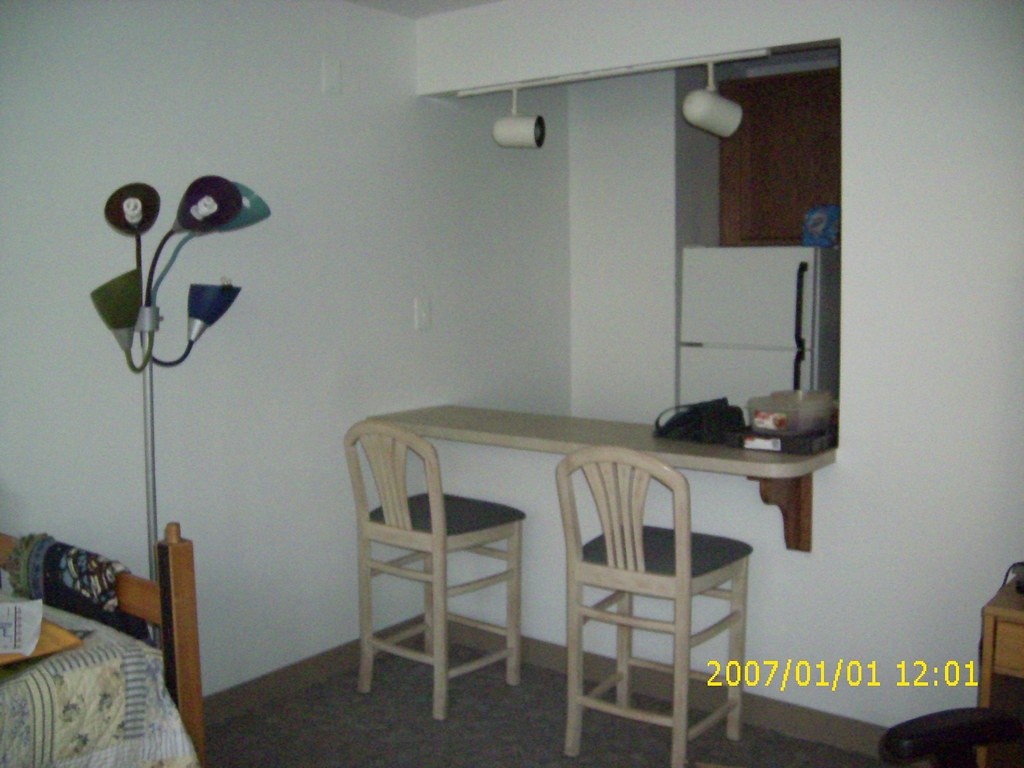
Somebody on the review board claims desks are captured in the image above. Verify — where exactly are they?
[977,576,1024,768]
[362,406,834,479]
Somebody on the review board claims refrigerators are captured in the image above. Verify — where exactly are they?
[679,246,839,414]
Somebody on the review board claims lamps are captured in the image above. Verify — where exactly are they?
[493,114,547,148]
[90,176,270,647]
[684,90,743,138]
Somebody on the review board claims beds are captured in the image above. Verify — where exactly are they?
[0,521,207,768]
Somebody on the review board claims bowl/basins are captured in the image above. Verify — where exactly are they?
[746,390,835,436]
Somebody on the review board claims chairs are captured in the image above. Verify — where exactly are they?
[341,419,528,723]
[554,446,754,768]
